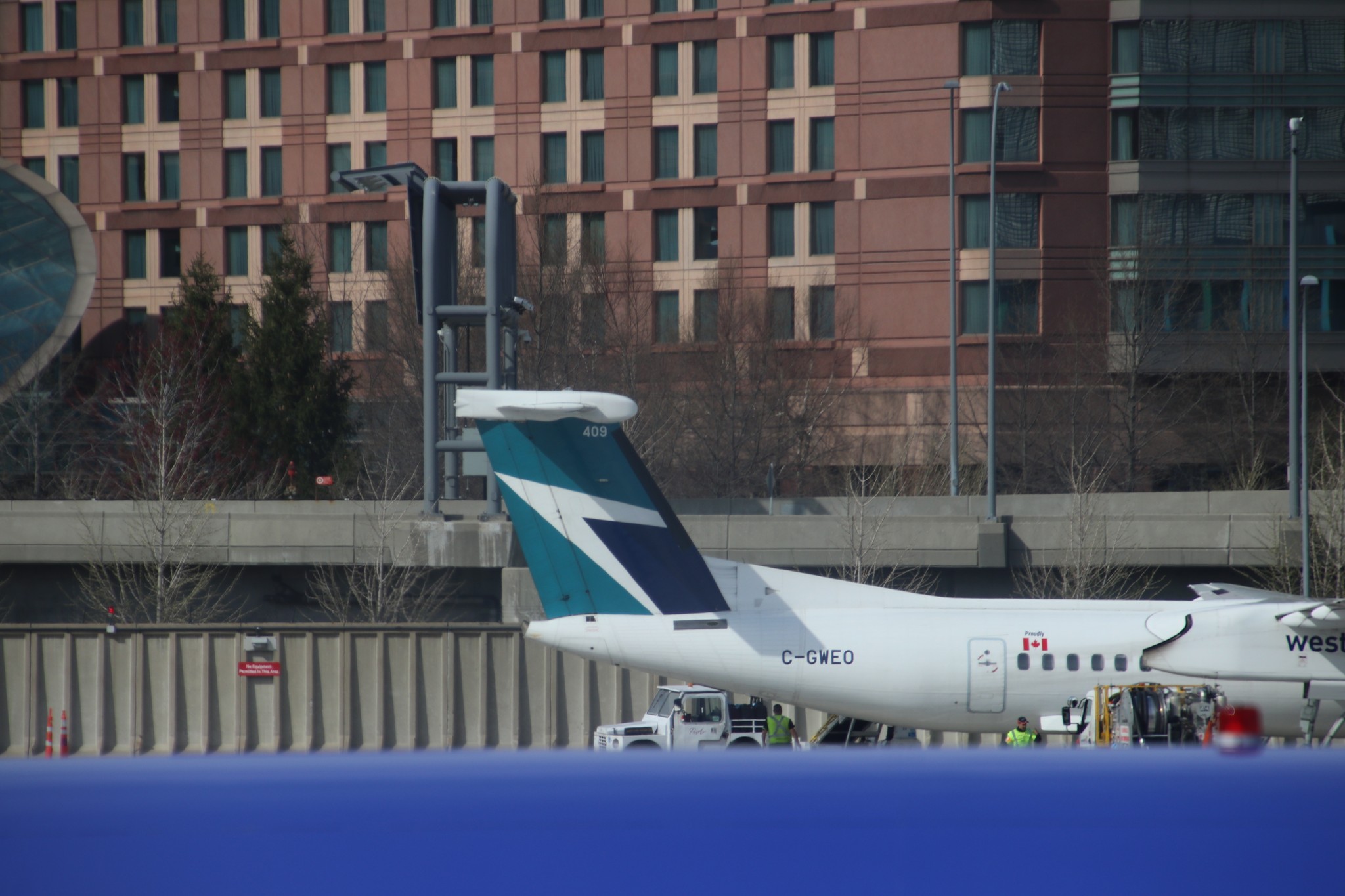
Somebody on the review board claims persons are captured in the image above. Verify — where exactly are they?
[761,702,803,750]
[1004,717,1042,750]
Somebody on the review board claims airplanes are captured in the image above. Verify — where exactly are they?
[454,390,1345,739]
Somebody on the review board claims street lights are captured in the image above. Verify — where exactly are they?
[945,81,961,496]
[1289,118,1302,517]
[987,82,1009,518]
[1300,275,1320,597]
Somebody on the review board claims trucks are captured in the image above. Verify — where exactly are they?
[1062,681,1227,747]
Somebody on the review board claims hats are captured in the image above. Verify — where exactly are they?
[1018,716,1029,725]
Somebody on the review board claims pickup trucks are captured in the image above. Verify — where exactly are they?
[594,683,767,752]
